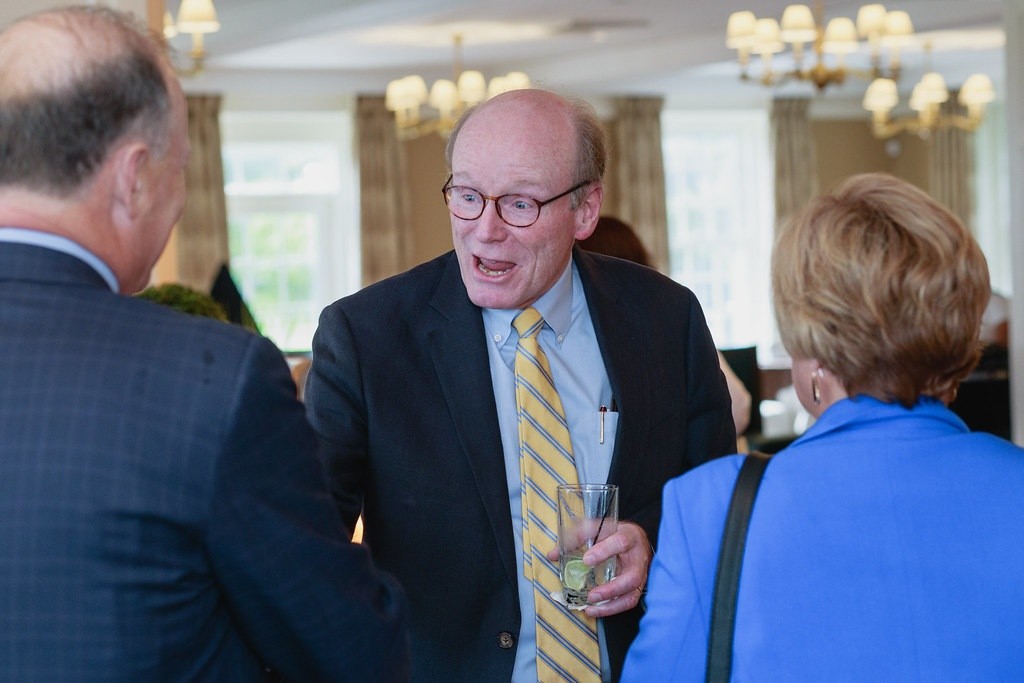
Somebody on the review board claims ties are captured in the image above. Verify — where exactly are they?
[512,307,602,683]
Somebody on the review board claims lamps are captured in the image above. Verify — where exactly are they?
[387,36,532,142]
[862,47,994,140]
[725,0,922,89]
[147,0,222,77]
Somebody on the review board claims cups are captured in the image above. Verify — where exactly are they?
[759,387,811,442]
[556,484,619,606]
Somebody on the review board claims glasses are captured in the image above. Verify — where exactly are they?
[442,175,596,228]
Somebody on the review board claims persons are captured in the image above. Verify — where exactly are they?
[621,172,1023,682]
[0,6,455,683]
[136,87,752,683]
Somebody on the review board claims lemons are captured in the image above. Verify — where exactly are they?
[563,559,592,591]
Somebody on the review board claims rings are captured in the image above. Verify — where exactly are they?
[638,586,644,598]
